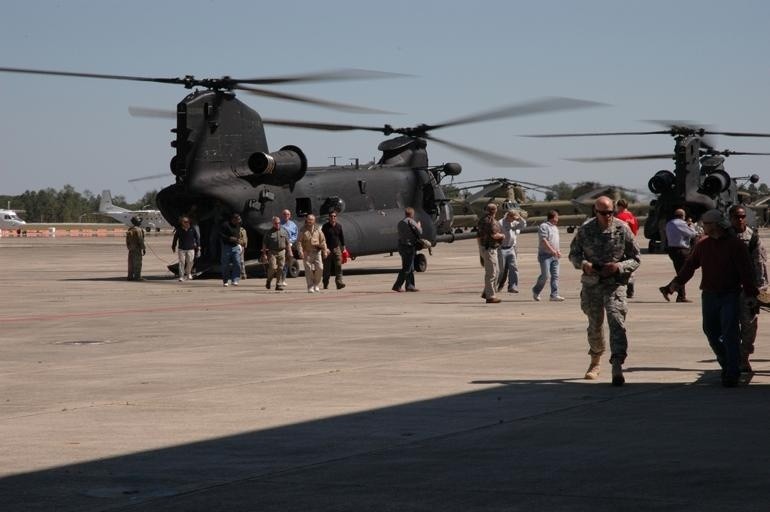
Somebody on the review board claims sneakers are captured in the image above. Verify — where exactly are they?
[265,281,285,292]
[308,286,320,293]
[532,288,540,301]
[550,295,565,302]
[481,291,501,304]
[738,357,753,374]
[720,366,740,388]
[337,283,345,289]
[508,288,519,293]
[178,273,193,282]
[405,288,420,293]
[585,355,603,380]
[611,359,625,385]
[659,286,671,301]
[676,299,694,303]
[224,275,246,287]
[392,287,405,293]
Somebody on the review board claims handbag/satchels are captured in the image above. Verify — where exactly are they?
[414,239,424,252]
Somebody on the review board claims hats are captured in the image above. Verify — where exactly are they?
[702,209,721,224]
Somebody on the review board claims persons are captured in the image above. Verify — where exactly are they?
[532,205,565,301]
[218,208,345,294]
[477,197,528,303]
[125,216,146,281]
[392,207,423,291]
[568,195,641,386]
[172,217,202,281]
[660,205,770,386]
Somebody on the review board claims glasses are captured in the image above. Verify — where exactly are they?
[596,209,615,216]
[729,214,746,219]
